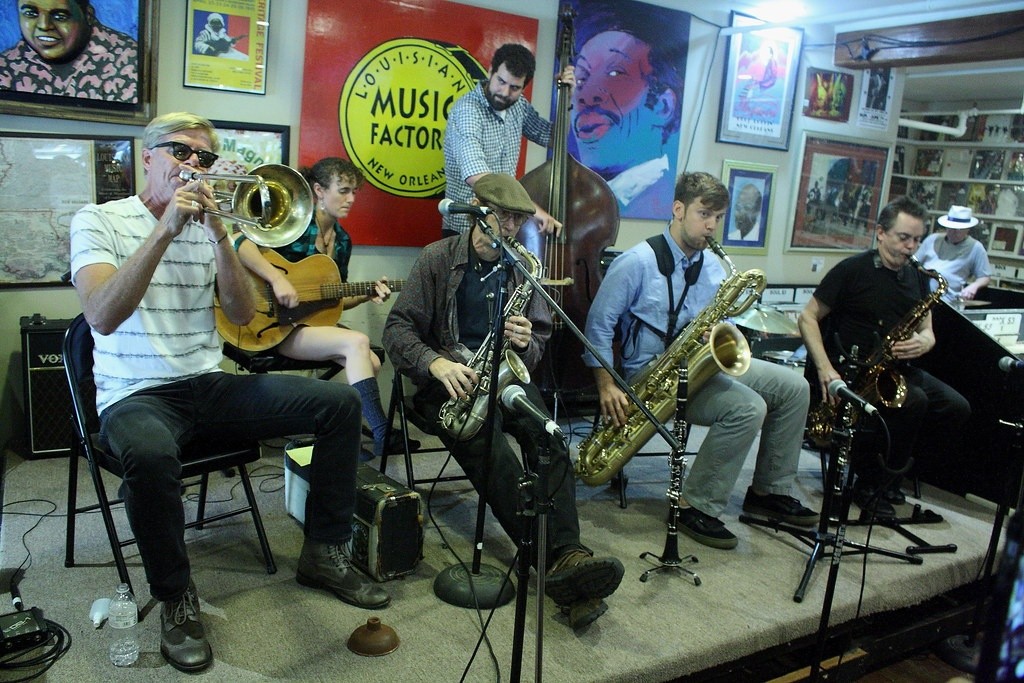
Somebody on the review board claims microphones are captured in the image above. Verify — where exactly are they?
[828,379,880,417]
[502,385,569,441]
[999,355,1024,372]
[438,199,495,215]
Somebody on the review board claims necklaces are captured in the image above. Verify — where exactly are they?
[315,216,334,256]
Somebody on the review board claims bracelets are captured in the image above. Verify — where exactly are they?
[208,232,227,245]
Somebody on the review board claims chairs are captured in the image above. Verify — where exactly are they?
[62,313,277,619]
[381,366,531,494]
[802,314,921,498]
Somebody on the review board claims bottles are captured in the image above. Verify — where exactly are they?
[90,598,111,628]
[108,583,139,667]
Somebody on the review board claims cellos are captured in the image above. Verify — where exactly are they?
[516,7,621,424]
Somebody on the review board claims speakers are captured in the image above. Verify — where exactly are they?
[20,316,81,459]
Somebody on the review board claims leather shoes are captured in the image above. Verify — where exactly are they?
[852,477,896,517]
[874,474,906,506]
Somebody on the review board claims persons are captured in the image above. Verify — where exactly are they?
[230,154,423,462]
[440,43,575,239]
[69,111,390,674]
[728,182,763,242]
[796,195,974,517]
[581,171,821,551]
[380,171,626,633]
[912,204,991,313]
[804,112,1024,248]
[193,12,248,59]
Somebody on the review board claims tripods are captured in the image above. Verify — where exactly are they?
[738,330,924,604]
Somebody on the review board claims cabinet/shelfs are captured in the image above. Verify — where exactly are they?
[889,139,1024,293]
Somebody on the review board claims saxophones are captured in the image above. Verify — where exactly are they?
[435,236,542,440]
[807,256,949,449]
[578,236,770,486]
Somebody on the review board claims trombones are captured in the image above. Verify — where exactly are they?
[181,163,313,247]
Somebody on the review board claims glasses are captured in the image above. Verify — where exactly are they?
[150,141,219,168]
[489,205,531,226]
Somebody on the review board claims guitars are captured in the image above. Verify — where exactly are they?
[215,249,406,351]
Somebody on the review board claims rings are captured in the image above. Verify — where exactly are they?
[191,200,199,207]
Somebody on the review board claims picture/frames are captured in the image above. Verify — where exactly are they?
[781,129,895,255]
[719,159,778,256]
[0,0,160,125]
[0,131,134,286]
[714,9,806,153]
[802,66,854,121]
[205,120,292,226]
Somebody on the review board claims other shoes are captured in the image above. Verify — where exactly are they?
[296,536,391,609]
[160,578,213,673]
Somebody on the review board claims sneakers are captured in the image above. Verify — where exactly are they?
[676,506,738,550]
[545,549,625,606]
[556,599,608,629]
[739,485,819,524]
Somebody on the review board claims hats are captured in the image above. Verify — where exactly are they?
[937,205,979,230]
[472,173,536,217]
